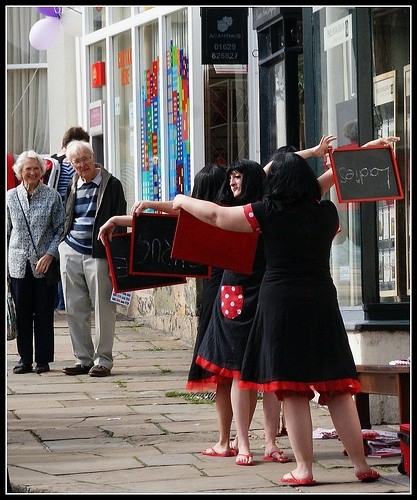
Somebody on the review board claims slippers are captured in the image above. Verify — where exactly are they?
[236,453,254,465]
[203,448,238,457]
[281,472,316,485]
[356,470,380,480]
[264,451,292,463]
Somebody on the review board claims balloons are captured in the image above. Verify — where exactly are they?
[36,7,62,19]
[29,16,62,51]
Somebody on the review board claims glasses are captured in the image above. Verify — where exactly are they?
[71,157,91,167]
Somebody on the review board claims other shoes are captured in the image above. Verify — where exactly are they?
[35,365,50,373]
[13,363,33,373]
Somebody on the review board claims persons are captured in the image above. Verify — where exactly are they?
[51,128,90,206]
[7,150,66,373]
[97,135,399,466]
[172,153,380,485]
[57,140,127,377]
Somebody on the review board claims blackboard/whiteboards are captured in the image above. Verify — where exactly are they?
[132,213,209,276]
[332,147,402,200]
[107,234,185,292]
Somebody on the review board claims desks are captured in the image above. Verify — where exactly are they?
[354,365,409,431]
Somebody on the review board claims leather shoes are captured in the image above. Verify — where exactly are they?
[89,366,110,376]
[62,364,94,375]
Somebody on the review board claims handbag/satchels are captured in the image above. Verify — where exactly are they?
[7,273,17,340]
[43,259,61,282]
